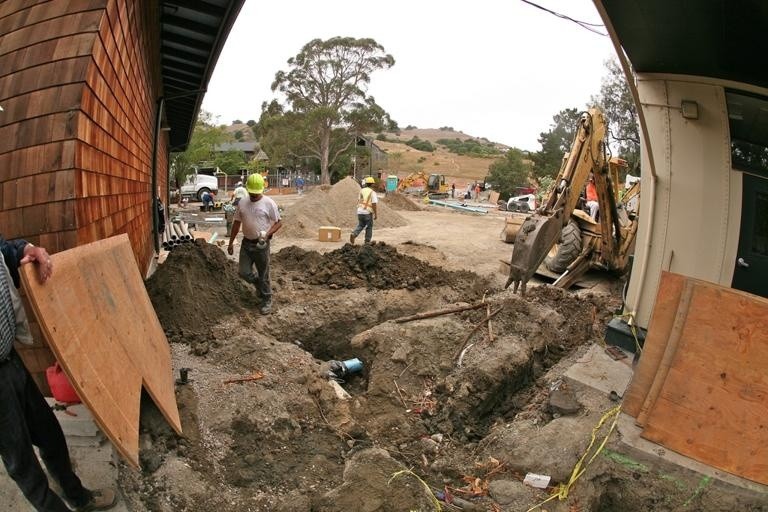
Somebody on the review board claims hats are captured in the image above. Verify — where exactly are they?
[237,180,243,186]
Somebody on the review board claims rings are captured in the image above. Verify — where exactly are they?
[46,259,51,269]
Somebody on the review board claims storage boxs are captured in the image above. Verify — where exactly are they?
[318,226,341,242]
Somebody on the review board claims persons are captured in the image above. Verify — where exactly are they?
[295,176,304,195]
[473,183,482,203]
[585,171,598,221]
[229,180,247,206]
[0,233,119,512]
[349,176,377,244]
[227,173,282,315]
[202,192,215,212]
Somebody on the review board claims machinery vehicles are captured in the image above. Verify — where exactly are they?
[501,107,641,296]
[396,171,449,200]
[170,174,218,202]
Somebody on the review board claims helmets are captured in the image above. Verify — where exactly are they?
[365,177,376,184]
[245,172,265,195]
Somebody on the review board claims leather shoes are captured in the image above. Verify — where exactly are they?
[260,297,272,315]
[69,487,118,512]
[254,272,264,298]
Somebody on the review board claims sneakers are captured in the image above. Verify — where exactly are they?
[349,233,355,244]
